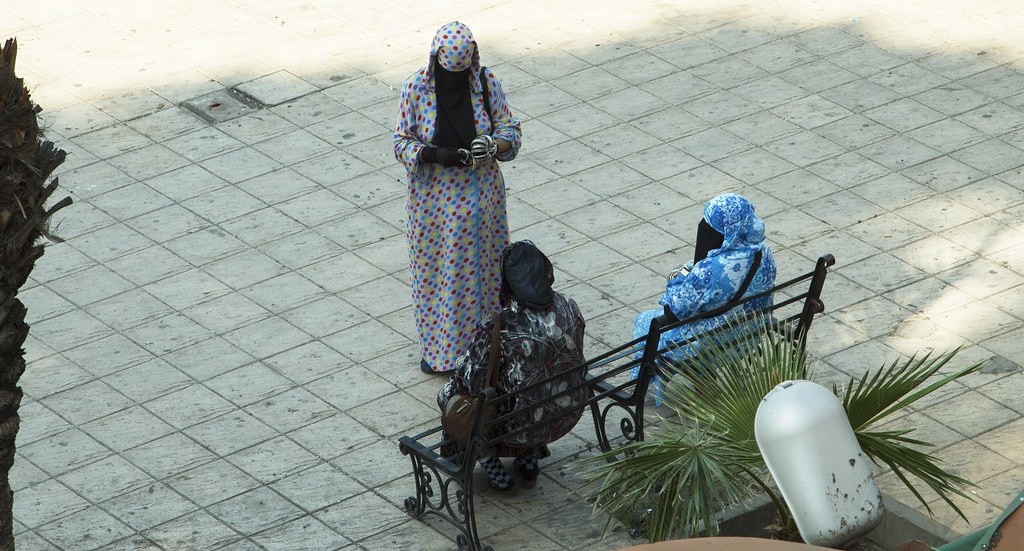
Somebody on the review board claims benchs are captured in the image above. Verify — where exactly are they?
[398,253,834,551]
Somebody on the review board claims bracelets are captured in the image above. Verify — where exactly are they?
[458,135,498,166]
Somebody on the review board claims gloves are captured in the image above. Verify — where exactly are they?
[421,147,471,167]
[473,142,500,159]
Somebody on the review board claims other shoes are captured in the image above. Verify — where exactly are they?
[420,357,435,374]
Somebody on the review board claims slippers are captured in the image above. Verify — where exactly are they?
[482,472,515,496]
[513,457,537,489]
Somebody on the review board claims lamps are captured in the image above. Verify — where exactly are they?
[754,378,886,549]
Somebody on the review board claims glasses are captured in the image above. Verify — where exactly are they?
[499,239,535,276]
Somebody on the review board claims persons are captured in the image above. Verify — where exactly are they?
[390,20,521,372]
[632,193,778,406]
[437,239,589,496]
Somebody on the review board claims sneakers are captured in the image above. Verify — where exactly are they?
[624,384,657,406]
